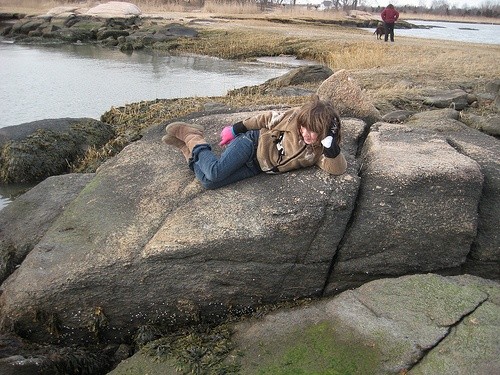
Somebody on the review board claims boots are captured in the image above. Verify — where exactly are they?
[162,134,191,162]
[166,121,207,153]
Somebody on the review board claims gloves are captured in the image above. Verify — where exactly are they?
[321,117,337,149]
[218,126,235,148]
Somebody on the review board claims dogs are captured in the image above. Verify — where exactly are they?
[373,27,391,40]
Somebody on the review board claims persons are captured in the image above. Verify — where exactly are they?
[160,99,347,190]
[380,3,399,42]
